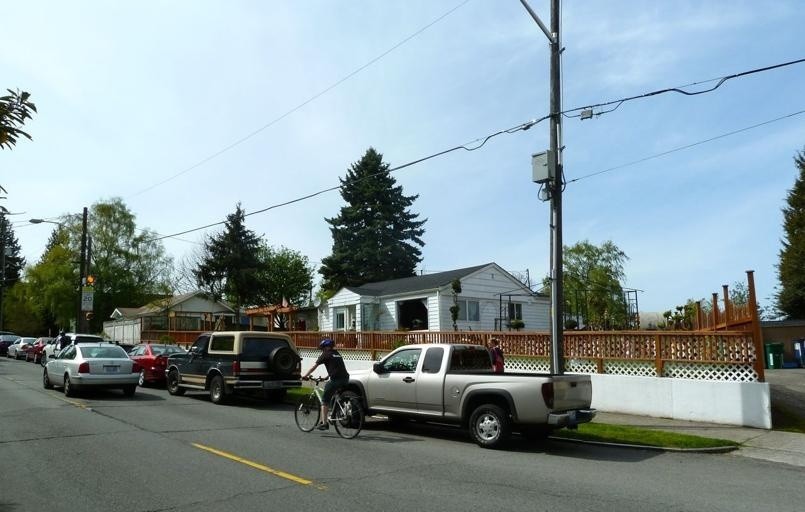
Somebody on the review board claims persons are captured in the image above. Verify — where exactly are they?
[486,338,505,373]
[303,337,350,430]
[54,330,67,351]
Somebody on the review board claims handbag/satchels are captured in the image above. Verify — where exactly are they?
[492,355,503,372]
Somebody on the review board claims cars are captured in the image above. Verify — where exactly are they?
[125,341,190,388]
[0,330,106,367]
[42,341,142,398]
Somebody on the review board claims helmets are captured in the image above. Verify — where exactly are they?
[319,339,334,350]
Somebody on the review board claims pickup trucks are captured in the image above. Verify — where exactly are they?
[330,341,599,452]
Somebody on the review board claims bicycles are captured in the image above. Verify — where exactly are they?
[294,371,367,440]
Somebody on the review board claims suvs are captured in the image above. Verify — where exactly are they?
[162,327,305,405]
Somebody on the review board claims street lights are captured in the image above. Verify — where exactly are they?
[28,218,93,333]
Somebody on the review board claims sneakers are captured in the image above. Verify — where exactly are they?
[314,421,329,430]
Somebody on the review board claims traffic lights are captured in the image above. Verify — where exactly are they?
[85,274,94,284]
